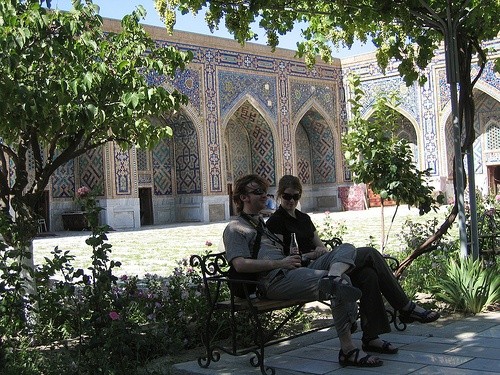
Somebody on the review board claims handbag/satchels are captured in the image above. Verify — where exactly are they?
[225,264,259,299]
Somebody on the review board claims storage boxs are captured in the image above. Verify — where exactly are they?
[367,186,396,206]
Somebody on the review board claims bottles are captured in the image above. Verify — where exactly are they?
[290,233,299,256]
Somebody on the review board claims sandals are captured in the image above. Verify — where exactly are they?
[338,347,384,368]
[317,274,363,303]
[360,335,399,355]
[398,300,441,324]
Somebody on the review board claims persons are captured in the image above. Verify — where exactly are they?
[264,194,280,210]
[222,173,384,367]
[265,175,440,355]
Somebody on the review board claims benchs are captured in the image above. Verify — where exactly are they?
[190,252,407,375]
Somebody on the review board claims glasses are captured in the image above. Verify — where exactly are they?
[281,191,302,201]
[247,187,268,196]
